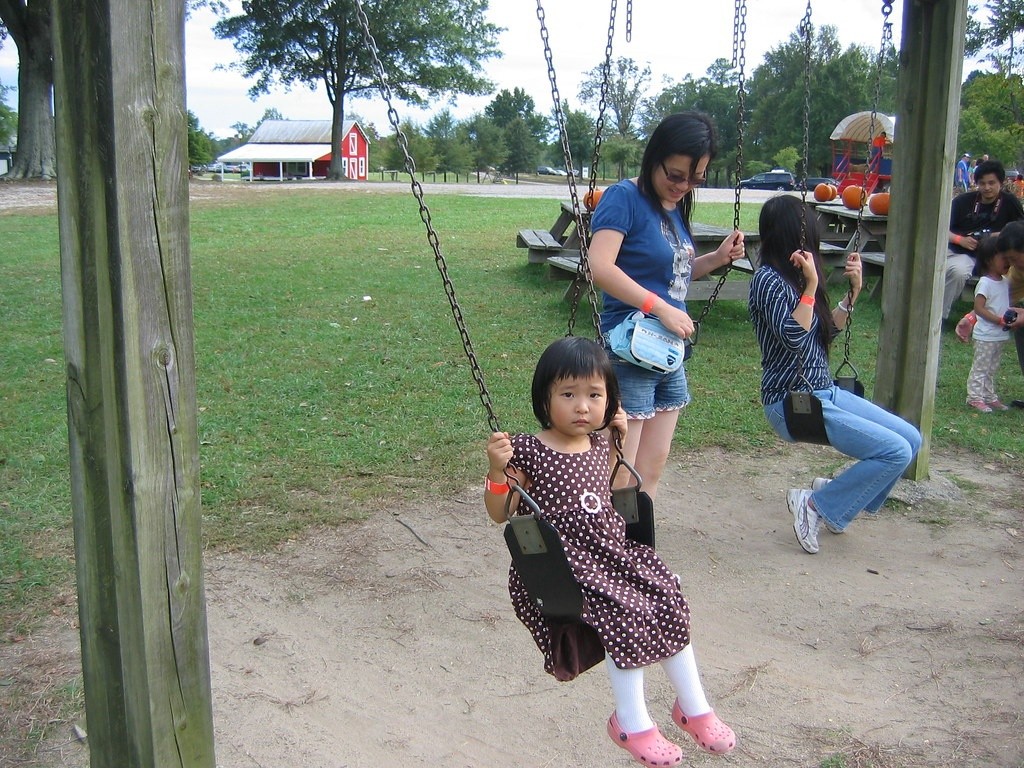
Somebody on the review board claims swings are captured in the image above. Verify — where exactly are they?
[353,1,658,622]
[564,0,897,447]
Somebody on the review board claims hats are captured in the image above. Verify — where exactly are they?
[962,153,971,158]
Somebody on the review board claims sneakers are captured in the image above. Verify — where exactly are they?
[986,399,1009,412]
[786,490,825,554]
[811,476,848,536]
[968,400,992,414]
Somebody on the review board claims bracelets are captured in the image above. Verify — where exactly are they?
[955,235,963,245]
[800,294,815,307]
[485,472,509,495]
[965,313,976,325]
[640,292,658,313]
[838,301,853,312]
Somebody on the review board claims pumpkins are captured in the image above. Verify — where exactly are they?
[814,183,837,202]
[842,184,867,210]
[869,189,889,215]
[583,186,604,211]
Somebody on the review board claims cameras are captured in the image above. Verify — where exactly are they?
[967,228,992,240]
[1001,310,1017,331]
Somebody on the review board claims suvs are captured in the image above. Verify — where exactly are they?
[536,166,557,175]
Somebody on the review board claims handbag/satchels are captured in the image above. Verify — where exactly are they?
[608,309,690,377]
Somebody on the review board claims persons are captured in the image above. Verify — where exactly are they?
[585,112,744,504]
[484,338,735,768]
[955,222,1024,413]
[941,162,1024,333]
[1013,174,1024,199]
[954,153,988,194]
[869,132,886,174]
[747,194,921,555]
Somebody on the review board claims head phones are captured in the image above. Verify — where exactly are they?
[971,191,1002,216]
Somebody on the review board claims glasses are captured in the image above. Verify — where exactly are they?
[659,158,708,187]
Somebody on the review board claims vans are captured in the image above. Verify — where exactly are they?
[738,171,796,192]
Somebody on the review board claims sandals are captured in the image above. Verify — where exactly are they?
[672,696,736,754]
[606,708,683,768]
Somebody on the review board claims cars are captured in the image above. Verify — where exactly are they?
[192,162,250,173]
[479,165,497,174]
[556,170,568,176]
[573,170,580,176]
[794,178,841,191]
